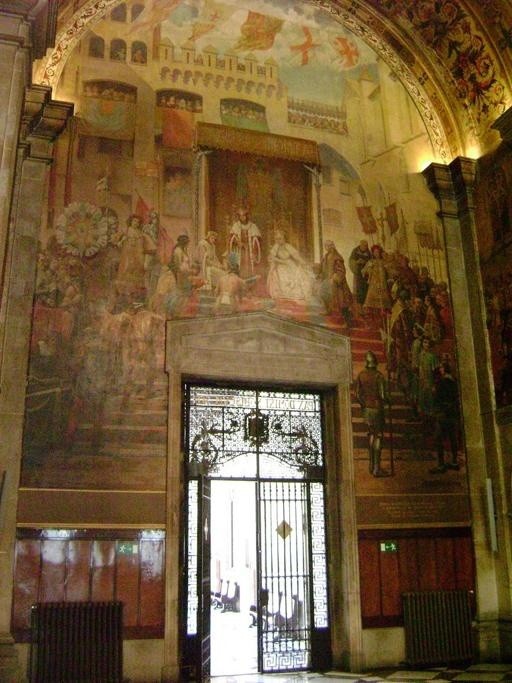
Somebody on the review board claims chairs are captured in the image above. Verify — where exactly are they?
[248,587,302,643]
[209,579,239,613]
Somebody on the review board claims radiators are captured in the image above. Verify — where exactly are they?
[401,590,477,670]
[27,601,124,681]
